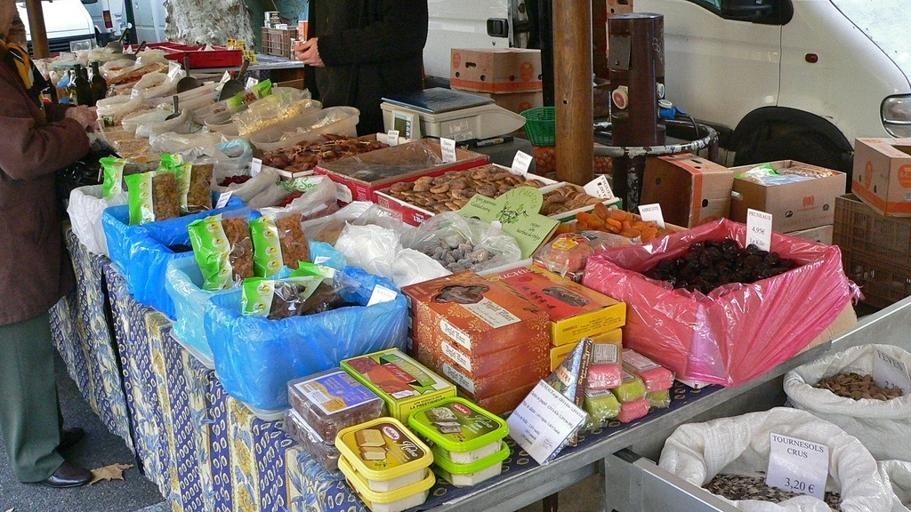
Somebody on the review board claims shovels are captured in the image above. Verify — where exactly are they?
[124,41,146,60]
[219,59,249,101]
[177,56,200,94]
[112,28,128,53]
[166,96,181,121]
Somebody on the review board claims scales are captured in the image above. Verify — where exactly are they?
[380,87,527,142]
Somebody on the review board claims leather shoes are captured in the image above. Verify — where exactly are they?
[58,427,86,448]
[40,460,93,488]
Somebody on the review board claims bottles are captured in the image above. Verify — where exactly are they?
[41,63,58,104]
[75,62,106,107]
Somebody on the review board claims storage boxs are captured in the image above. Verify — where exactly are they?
[125,42,361,165]
[204,267,407,421]
[69,184,129,257]
[533,211,686,282]
[315,138,489,201]
[129,205,261,319]
[164,240,346,360]
[104,190,242,273]
[449,50,543,112]
[374,162,558,228]
[538,181,622,222]
[268,132,411,180]
[584,218,859,388]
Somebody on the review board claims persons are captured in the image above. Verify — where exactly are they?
[292,0,429,141]
[1,1,101,488]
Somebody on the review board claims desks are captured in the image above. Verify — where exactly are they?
[43,201,829,512]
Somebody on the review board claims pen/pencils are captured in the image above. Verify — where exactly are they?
[475,136,514,148]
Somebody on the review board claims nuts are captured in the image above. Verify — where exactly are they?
[123,101,800,319]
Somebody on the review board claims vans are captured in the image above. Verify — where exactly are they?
[421,2,910,192]
[14,0,168,59]
